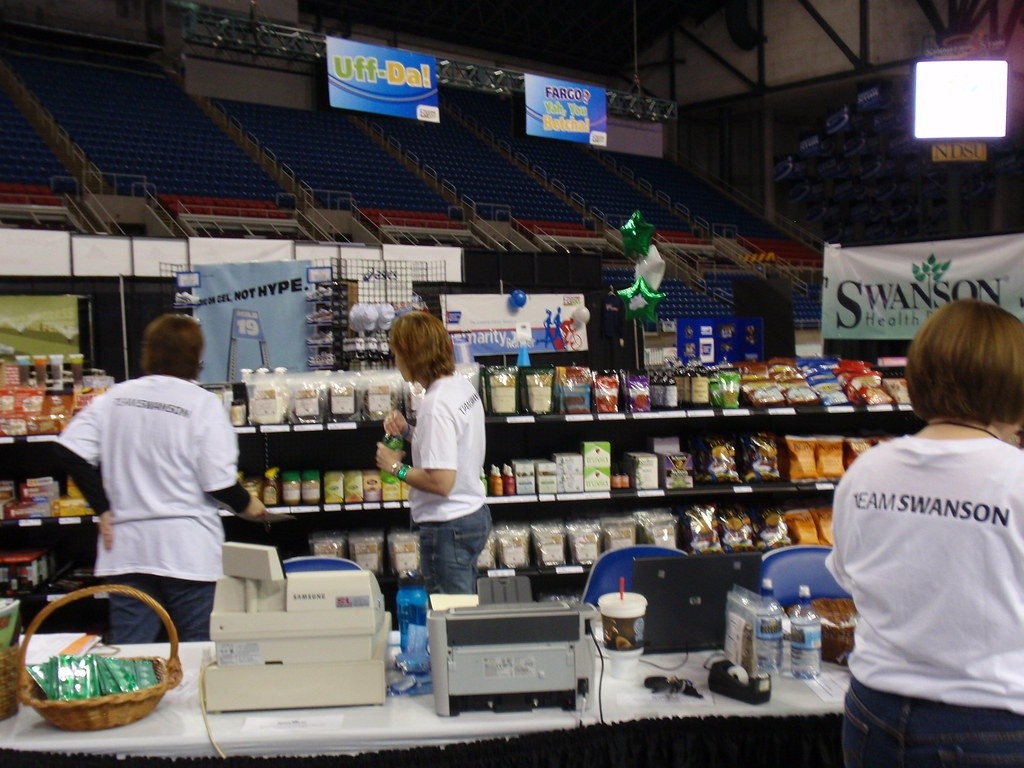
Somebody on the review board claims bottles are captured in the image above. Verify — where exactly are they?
[397,572,429,651]
[223,383,247,426]
[648,357,740,410]
[789,586,819,680]
[753,578,784,678]
[612,472,630,488]
[380,433,404,484]
[480,463,516,496]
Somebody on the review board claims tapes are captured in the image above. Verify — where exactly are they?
[727,665,749,684]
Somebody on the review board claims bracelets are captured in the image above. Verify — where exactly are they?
[405,424,411,438]
[398,465,410,481]
[391,461,400,475]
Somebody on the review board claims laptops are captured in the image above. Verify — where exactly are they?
[632,552,763,653]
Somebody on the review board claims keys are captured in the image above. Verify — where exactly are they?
[643,676,704,700]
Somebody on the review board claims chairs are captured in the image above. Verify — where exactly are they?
[0,6,830,331]
[757,543,853,603]
[581,542,687,605]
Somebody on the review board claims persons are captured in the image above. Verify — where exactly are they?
[825,298,1024,768]
[54,315,269,645]
[376,311,493,613]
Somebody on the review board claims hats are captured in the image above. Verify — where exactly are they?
[363,304,379,331]
[378,304,395,330]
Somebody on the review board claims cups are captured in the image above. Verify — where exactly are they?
[596,593,648,651]
[604,648,642,679]
[16,354,84,386]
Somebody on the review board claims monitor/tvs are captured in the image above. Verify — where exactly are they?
[910,58,1011,140]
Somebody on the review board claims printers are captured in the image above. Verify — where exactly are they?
[426,576,597,717]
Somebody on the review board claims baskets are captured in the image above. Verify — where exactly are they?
[0,643,20,722]
[16,584,183,731]
[792,598,859,667]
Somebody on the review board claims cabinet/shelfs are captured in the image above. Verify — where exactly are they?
[0,358,922,633]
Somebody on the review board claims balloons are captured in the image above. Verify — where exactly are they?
[573,305,590,323]
[617,276,664,323]
[634,244,666,290]
[618,209,654,258]
[511,290,527,309]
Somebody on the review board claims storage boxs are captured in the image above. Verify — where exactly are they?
[511,441,610,492]
[626,436,694,489]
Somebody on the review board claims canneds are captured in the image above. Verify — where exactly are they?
[280,469,322,504]
